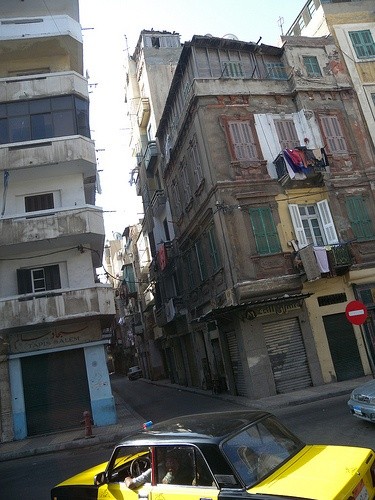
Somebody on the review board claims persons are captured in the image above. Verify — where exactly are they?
[122,447,218,491]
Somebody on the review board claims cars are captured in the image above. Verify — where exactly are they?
[51,410,375,500]
[346,377,375,423]
[127,366,143,381]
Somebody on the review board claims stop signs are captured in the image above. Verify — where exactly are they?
[345,300,368,325]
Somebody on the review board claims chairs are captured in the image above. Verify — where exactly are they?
[164,453,190,484]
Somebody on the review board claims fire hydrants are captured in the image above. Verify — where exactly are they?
[80,407,94,438]
